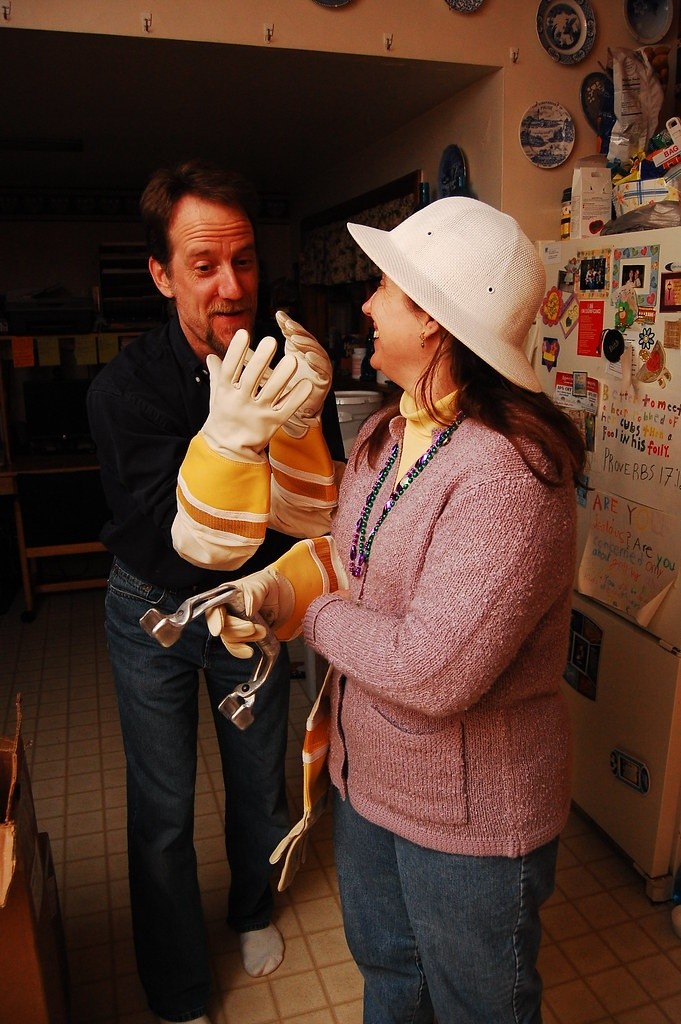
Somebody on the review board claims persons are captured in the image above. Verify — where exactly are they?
[207,195,587,1024]
[88,158,348,1024]
[626,270,641,288]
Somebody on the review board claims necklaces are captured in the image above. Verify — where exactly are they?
[349,416,469,577]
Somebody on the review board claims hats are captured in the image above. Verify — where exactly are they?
[346,196,543,394]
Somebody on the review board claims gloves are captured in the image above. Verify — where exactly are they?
[205,536,349,659]
[171,327,315,572]
[242,310,338,539]
[269,665,338,892]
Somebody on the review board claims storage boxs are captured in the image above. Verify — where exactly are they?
[0,691,69,1024]
[570,144,680,241]
